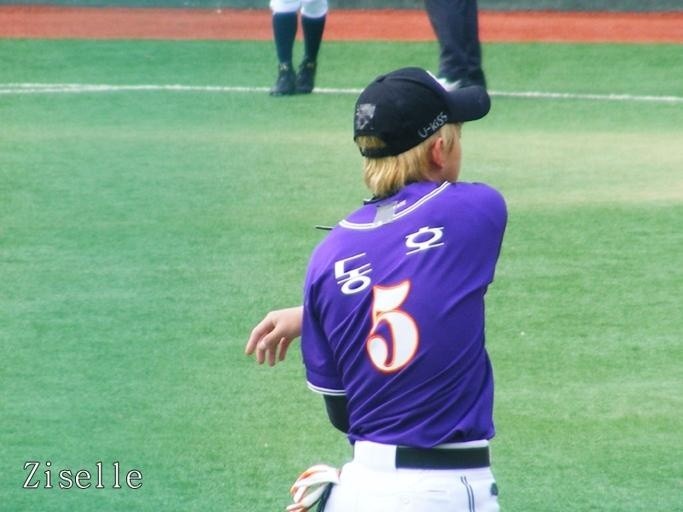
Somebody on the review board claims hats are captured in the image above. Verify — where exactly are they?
[353,66,491,158]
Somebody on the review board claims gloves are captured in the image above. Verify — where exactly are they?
[291,465,339,502]
[285,482,331,512]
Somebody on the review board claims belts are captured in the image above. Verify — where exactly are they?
[395,446,491,471]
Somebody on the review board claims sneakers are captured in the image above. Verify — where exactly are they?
[295,58,316,93]
[270,62,295,96]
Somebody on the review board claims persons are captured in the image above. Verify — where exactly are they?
[263,0,329,100]
[422,0,488,94]
[242,66,511,509]
